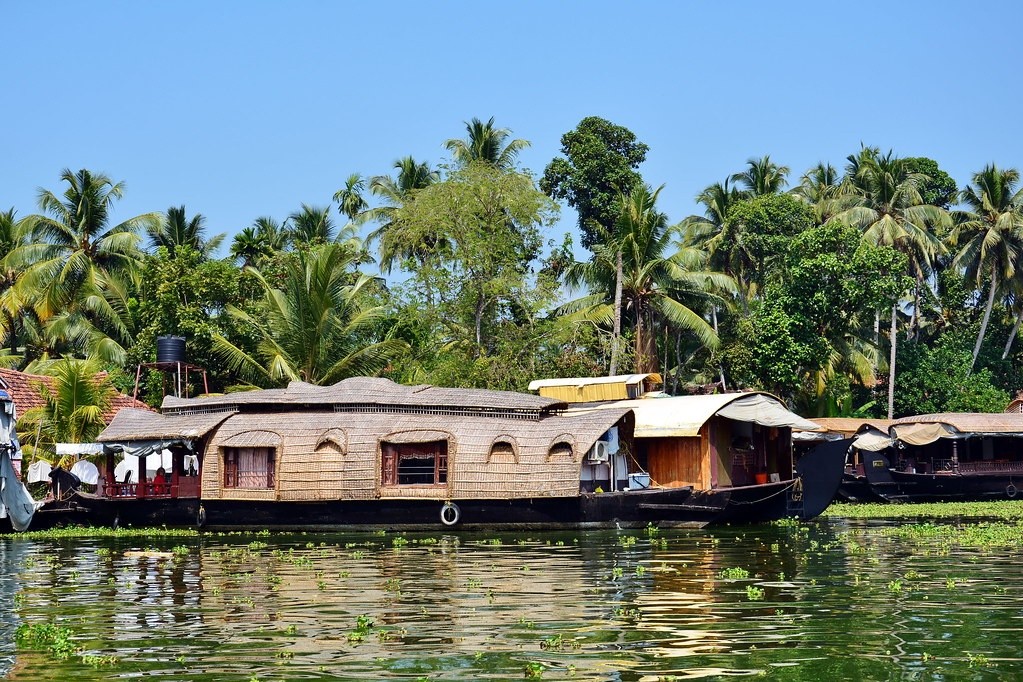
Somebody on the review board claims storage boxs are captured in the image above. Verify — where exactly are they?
[628,473,650,490]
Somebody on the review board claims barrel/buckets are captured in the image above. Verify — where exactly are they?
[756,473,768,485]
[770,472,779,482]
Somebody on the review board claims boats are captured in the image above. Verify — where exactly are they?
[0,339,1022,531]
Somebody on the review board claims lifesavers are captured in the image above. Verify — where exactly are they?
[440,504,461,525]
[891,496,908,505]
[1005,485,1018,498]
[902,488,914,497]
[196,510,206,528]
[910,497,919,504]
[112,518,119,530]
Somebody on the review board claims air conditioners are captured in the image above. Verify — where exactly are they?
[588,441,609,461]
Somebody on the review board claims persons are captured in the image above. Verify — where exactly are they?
[152,466,168,494]
